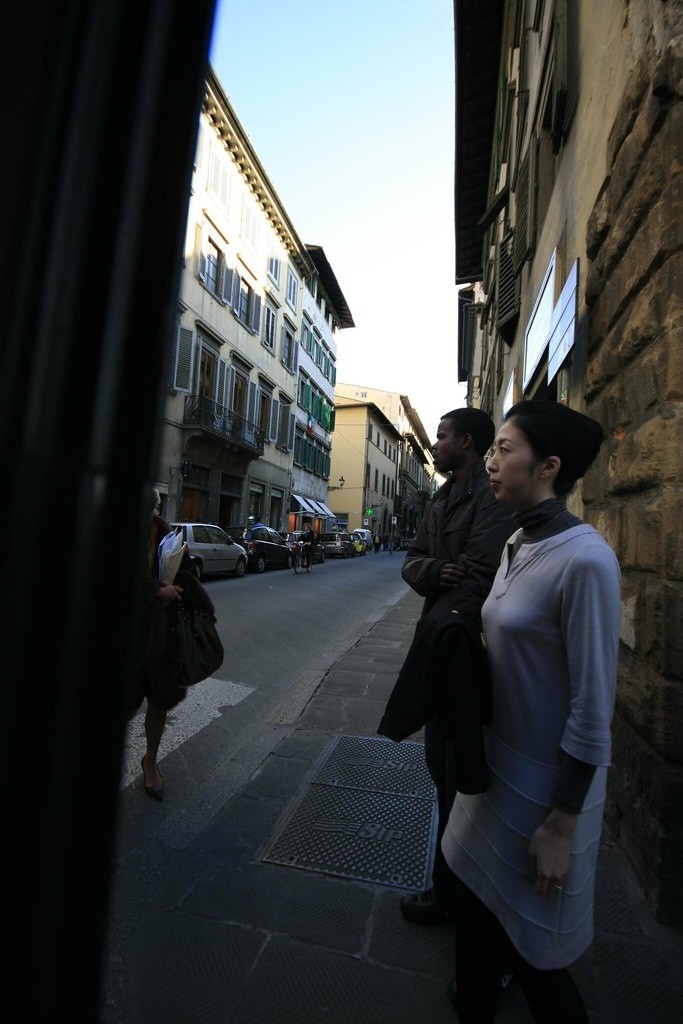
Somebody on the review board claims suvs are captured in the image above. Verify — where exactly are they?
[277,531,325,563]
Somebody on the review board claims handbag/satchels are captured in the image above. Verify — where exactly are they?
[169,602,223,687]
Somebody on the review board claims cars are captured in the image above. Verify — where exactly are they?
[221,526,294,573]
[171,523,248,581]
[349,533,367,556]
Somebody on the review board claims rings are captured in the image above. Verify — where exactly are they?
[553,884,563,890]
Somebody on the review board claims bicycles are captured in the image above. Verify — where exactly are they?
[293,541,312,574]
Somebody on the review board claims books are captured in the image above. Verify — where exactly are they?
[158,526,188,586]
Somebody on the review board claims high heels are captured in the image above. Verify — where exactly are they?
[141,756,164,801]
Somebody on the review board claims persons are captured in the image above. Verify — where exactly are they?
[128,485,192,804]
[397,407,519,929]
[387,531,396,555]
[245,516,267,541]
[297,522,314,568]
[439,398,623,1024]
[373,531,382,554]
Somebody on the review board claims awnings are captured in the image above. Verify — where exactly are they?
[290,493,337,522]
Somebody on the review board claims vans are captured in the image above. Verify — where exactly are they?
[322,532,355,558]
[353,528,372,550]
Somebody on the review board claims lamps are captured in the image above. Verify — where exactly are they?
[327,476,345,491]
[170,460,191,478]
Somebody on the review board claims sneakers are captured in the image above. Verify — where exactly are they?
[400,884,457,927]
[448,980,458,1010]
